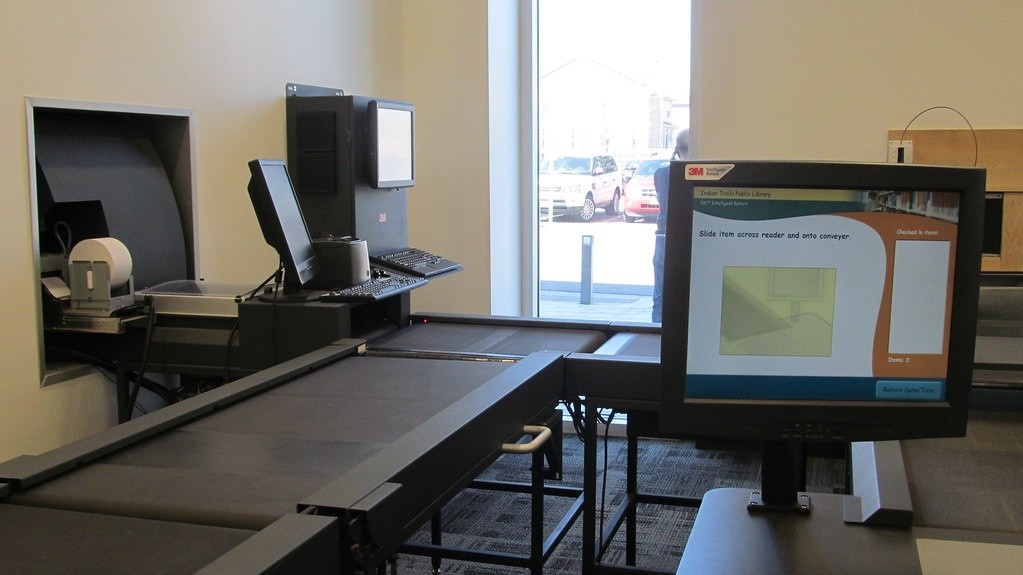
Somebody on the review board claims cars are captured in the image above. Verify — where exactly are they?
[621,160,641,183]
[624,158,672,223]
[539,153,623,222]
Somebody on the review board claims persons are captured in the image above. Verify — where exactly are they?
[652,129,688,323]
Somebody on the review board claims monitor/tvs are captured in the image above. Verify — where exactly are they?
[661,160,987,514]
[365,100,417,189]
[247,160,329,304]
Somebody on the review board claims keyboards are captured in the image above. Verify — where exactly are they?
[320,277,428,303]
[378,248,459,278]
